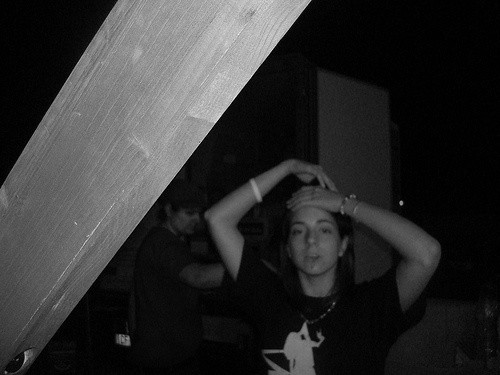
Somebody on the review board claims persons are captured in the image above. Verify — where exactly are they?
[127,186,226,355]
[205,159,440,375]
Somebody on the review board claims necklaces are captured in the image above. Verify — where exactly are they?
[300,297,338,324]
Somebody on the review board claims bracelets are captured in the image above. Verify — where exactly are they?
[340,192,357,215]
[250,178,263,203]
[353,200,362,216]
